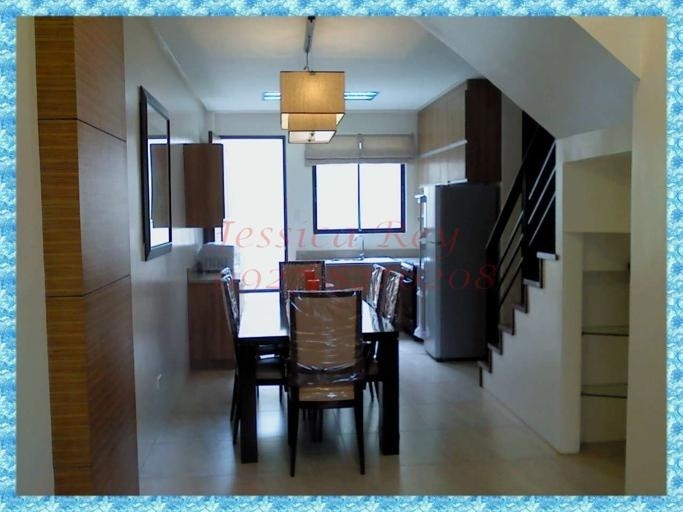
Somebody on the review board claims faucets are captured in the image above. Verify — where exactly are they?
[352,232,366,258]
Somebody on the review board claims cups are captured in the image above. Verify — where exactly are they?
[304,269,322,291]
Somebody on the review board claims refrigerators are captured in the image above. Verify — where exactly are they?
[420,182,500,362]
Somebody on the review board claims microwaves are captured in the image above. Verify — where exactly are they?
[200,242,234,273]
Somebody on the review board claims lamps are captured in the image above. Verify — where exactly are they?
[278,18,347,145]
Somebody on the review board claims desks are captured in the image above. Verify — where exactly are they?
[191,265,240,368]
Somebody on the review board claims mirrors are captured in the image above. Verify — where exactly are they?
[140,87,173,262]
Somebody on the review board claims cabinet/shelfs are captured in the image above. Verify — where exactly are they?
[413,79,502,187]
